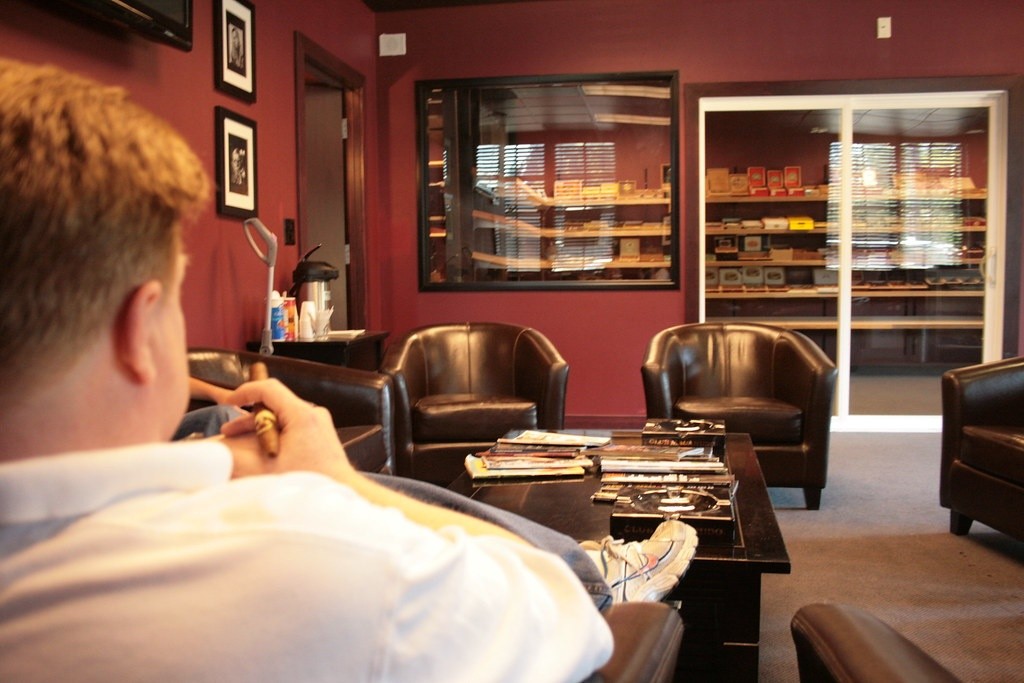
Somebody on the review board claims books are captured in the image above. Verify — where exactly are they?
[464,429,611,479]
[581,443,739,501]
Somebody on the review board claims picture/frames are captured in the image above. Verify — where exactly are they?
[214,105,258,220]
[214,0,256,103]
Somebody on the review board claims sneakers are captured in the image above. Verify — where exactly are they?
[579,520,699,606]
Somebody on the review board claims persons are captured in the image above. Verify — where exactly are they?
[171,376,253,441]
[0,55,698,683]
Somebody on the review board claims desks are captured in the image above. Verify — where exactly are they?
[437,426,793,682]
[249,329,391,368]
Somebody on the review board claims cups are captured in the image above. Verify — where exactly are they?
[300,301,317,339]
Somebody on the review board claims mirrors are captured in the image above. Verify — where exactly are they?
[413,66,679,292]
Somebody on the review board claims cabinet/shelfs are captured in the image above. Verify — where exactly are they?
[433,169,673,278]
[708,181,987,341]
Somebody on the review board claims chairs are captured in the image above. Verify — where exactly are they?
[639,320,839,510]
[378,321,571,484]
[185,347,398,484]
[940,355,1023,554]
